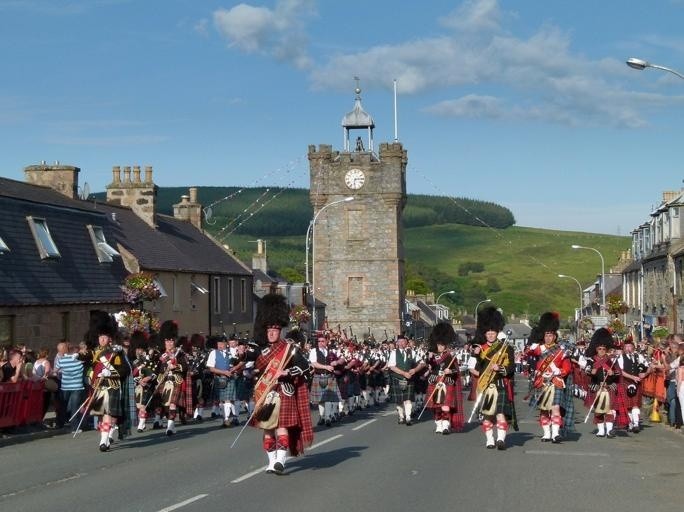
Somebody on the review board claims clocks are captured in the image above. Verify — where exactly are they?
[344,167,367,192]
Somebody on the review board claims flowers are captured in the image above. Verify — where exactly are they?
[606,293,629,316]
[113,308,161,338]
[118,269,162,304]
[608,318,629,338]
[292,302,312,328]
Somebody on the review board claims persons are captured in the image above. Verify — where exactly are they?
[243,325,314,475]
[467,326,517,450]
[514,326,684,442]
[1,331,260,451]
[300,332,471,434]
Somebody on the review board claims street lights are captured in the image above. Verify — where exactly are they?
[304,197,354,293]
[434,291,455,323]
[474,298,492,328]
[570,243,607,314]
[557,274,584,321]
[626,58,683,83]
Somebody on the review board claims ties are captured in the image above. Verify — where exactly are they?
[629,355,632,360]
[402,351,404,361]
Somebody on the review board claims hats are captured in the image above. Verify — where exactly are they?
[429,307,614,357]
[85,294,306,350]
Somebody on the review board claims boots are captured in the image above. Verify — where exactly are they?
[574,388,640,438]
[265,449,276,471]
[551,424,561,443]
[99,401,254,451]
[274,449,287,473]
[485,428,495,449]
[496,428,506,450]
[540,424,551,442]
[317,375,470,425]
[441,419,450,435]
[434,419,442,432]
